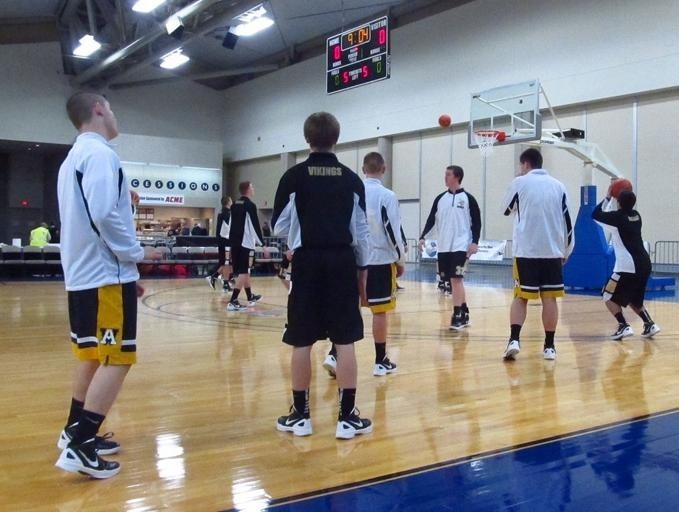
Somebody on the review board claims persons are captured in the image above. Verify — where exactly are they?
[227,180,268,311]
[418,164,481,329]
[54,91,162,480]
[204,194,235,293]
[130,191,142,215]
[29,222,52,248]
[591,183,659,340]
[500,148,575,360]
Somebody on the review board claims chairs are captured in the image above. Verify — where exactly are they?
[0,235,286,279]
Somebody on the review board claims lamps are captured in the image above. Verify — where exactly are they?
[167,14,185,41]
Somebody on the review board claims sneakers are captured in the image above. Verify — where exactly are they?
[336,408,372,440]
[641,323,660,337]
[544,344,557,361]
[374,355,397,376]
[611,325,632,340]
[324,351,336,378]
[276,406,312,437]
[206,273,263,310]
[450,310,470,329]
[502,340,520,360]
[438,280,452,294]
[55,438,120,478]
[57,422,121,455]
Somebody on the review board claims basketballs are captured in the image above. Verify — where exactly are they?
[608,179,633,196]
[438,115,451,127]
[427,241,437,257]
[130,192,139,205]
